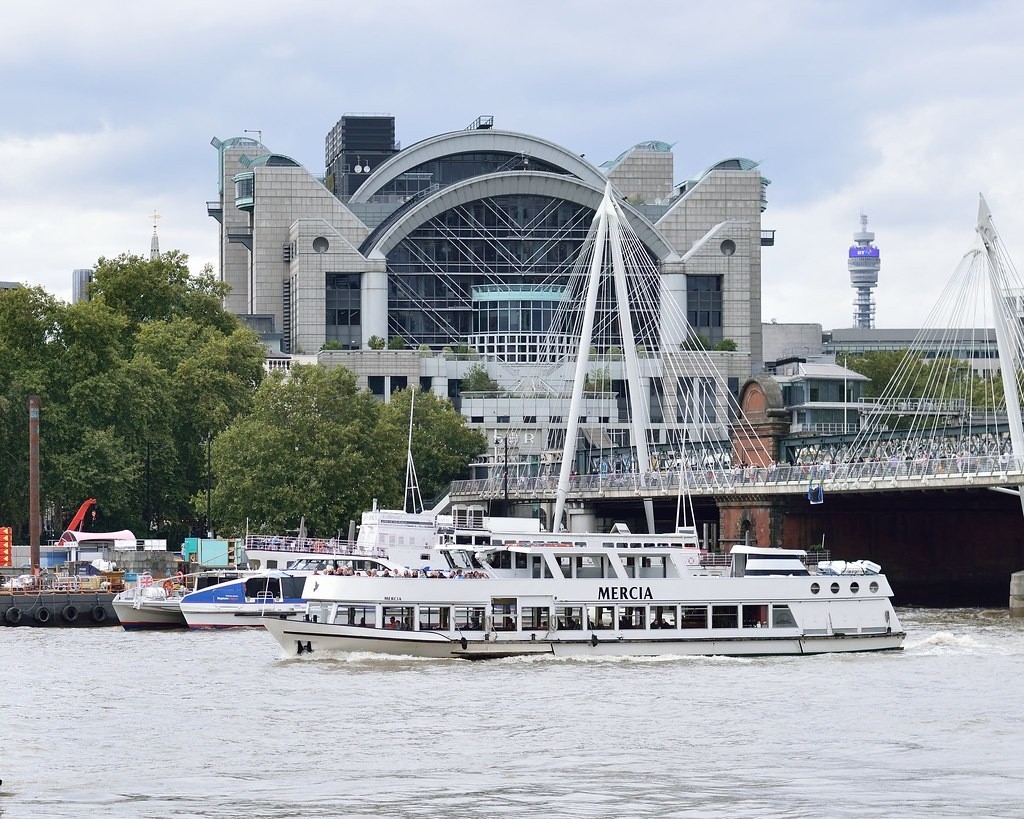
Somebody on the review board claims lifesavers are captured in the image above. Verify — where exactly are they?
[314,541,324,551]
[93,605,106,621]
[5,607,23,624]
[163,580,174,596]
[176,571,183,581]
[61,605,78,621]
[35,606,51,624]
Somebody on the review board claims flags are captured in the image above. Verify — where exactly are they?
[140,576,153,588]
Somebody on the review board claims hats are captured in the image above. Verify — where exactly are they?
[390,617,395,619]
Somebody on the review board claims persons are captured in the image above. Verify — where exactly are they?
[388,616,400,629]
[359,618,366,627]
[506,618,515,630]
[596,448,795,487]
[249,535,336,554]
[661,619,669,629]
[486,554,493,565]
[314,565,488,579]
[795,452,1014,479]
[651,620,659,629]
[571,619,580,630]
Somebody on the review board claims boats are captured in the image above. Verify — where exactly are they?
[112,551,454,633]
[261,385,907,661]
